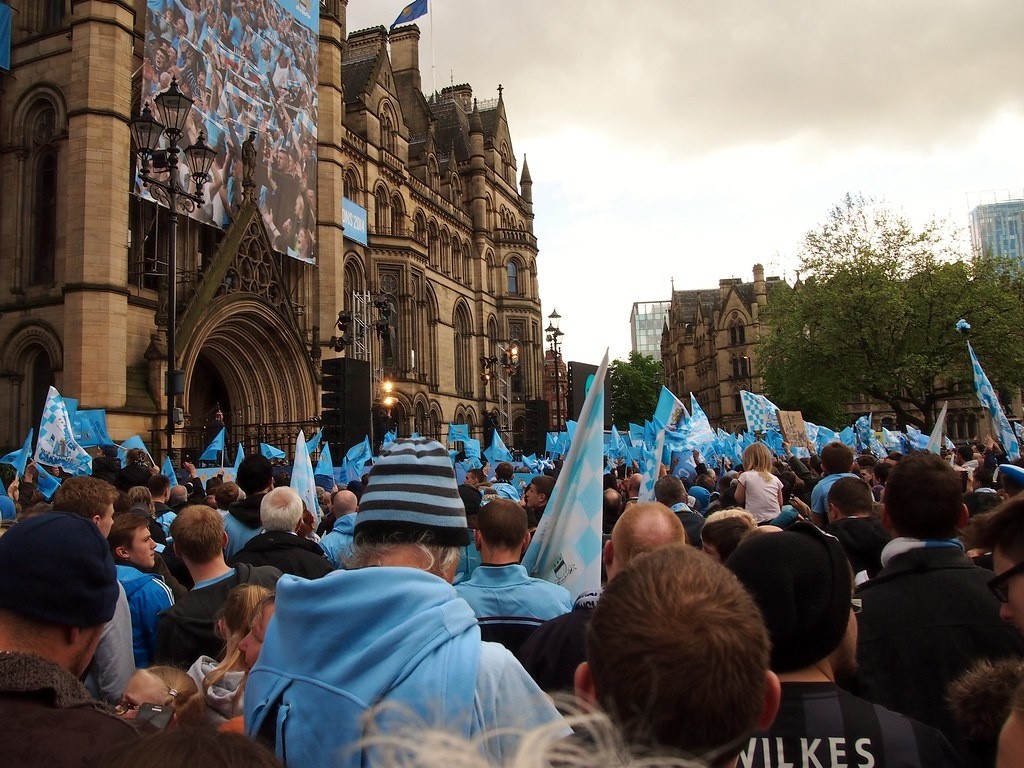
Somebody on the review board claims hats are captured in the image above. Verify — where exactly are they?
[457,483,483,515]
[351,435,471,546]
[732,520,851,672]
[1,510,118,626]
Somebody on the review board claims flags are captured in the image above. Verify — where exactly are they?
[387,0,429,29]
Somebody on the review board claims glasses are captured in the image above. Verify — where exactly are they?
[851,598,863,614]
[989,563,1024,603]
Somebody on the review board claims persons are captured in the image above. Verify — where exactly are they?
[2,328,1022,768]
[137,1,324,269]
